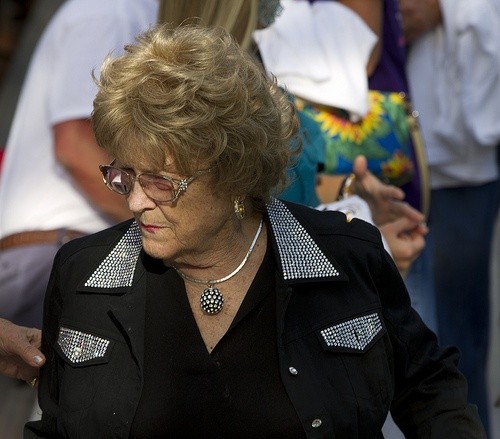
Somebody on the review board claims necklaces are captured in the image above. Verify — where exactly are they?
[162,216,265,314]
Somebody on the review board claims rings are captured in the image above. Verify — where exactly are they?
[31,376,38,386]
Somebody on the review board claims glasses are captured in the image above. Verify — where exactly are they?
[99,158,215,203]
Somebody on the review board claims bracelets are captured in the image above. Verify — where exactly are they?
[336,173,356,200]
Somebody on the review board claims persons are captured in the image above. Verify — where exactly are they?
[1,0,500,439]
